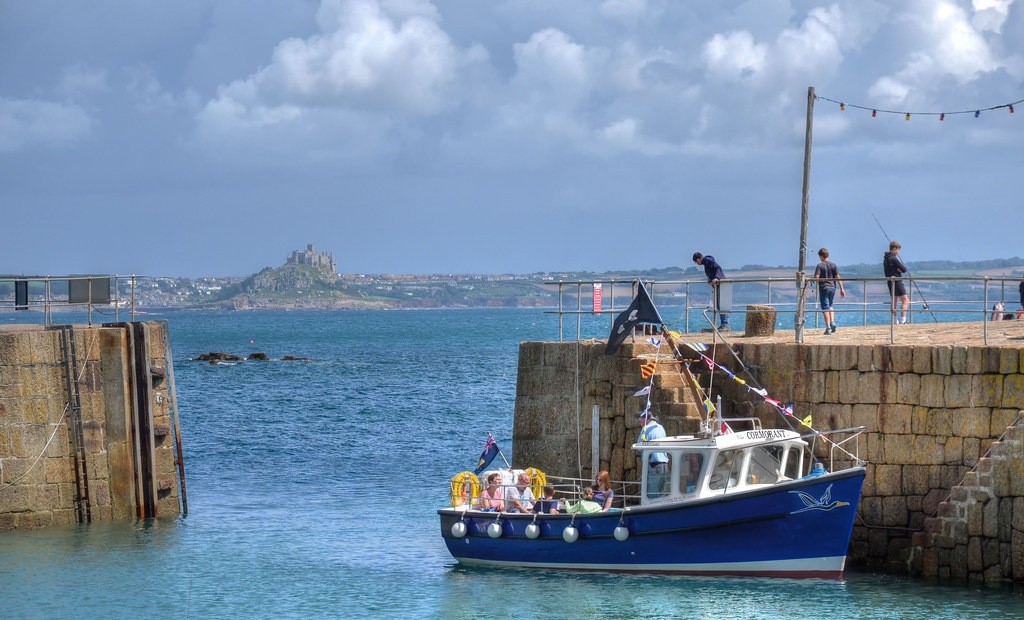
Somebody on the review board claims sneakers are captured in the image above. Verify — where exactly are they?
[718,323,730,331]
[830,321,837,333]
[823,327,832,335]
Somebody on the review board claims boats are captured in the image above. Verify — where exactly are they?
[435,418,871,586]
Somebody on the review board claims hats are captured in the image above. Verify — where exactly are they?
[639,412,659,421]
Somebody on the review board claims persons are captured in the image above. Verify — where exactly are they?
[813,247,845,335]
[560,487,603,514]
[506,473,537,513]
[590,470,614,512]
[693,251,731,332]
[637,413,669,496]
[883,240,909,325]
[480,473,503,513]
[530,486,559,514]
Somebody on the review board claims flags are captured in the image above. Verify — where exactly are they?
[633,326,814,429]
[474,434,501,476]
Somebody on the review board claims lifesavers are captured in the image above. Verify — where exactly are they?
[526,468,547,499]
[452,472,481,506]
